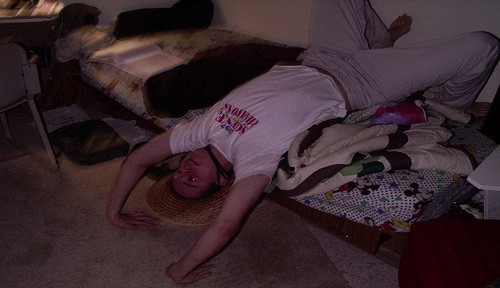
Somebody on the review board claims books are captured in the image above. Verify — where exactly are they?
[87,37,186,79]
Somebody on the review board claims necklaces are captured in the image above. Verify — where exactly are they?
[205,144,231,180]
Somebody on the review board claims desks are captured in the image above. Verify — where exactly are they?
[466,145,499,222]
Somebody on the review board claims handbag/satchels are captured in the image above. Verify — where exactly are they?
[48,119,129,165]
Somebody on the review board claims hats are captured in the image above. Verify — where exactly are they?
[144,171,236,226]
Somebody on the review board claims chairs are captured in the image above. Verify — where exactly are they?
[2,43,59,173]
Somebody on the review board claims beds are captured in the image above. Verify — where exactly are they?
[75,19,499,254]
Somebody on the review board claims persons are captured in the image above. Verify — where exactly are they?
[105,0,500,285]
